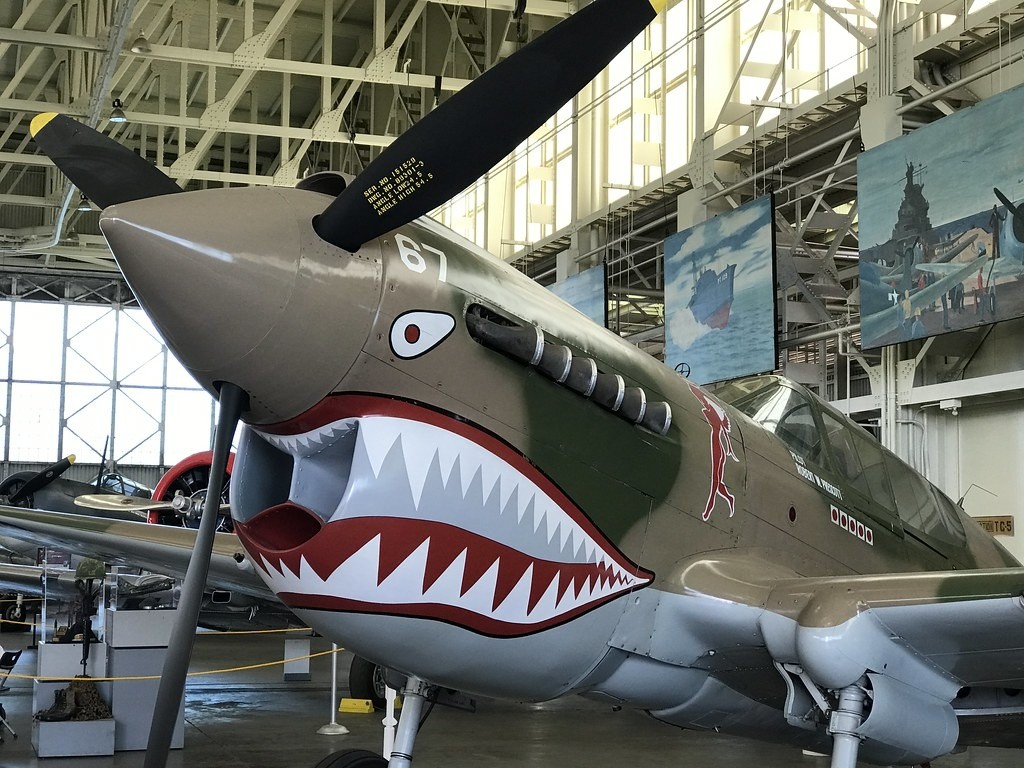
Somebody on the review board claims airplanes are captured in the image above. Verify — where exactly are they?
[0,0,1024,768]
